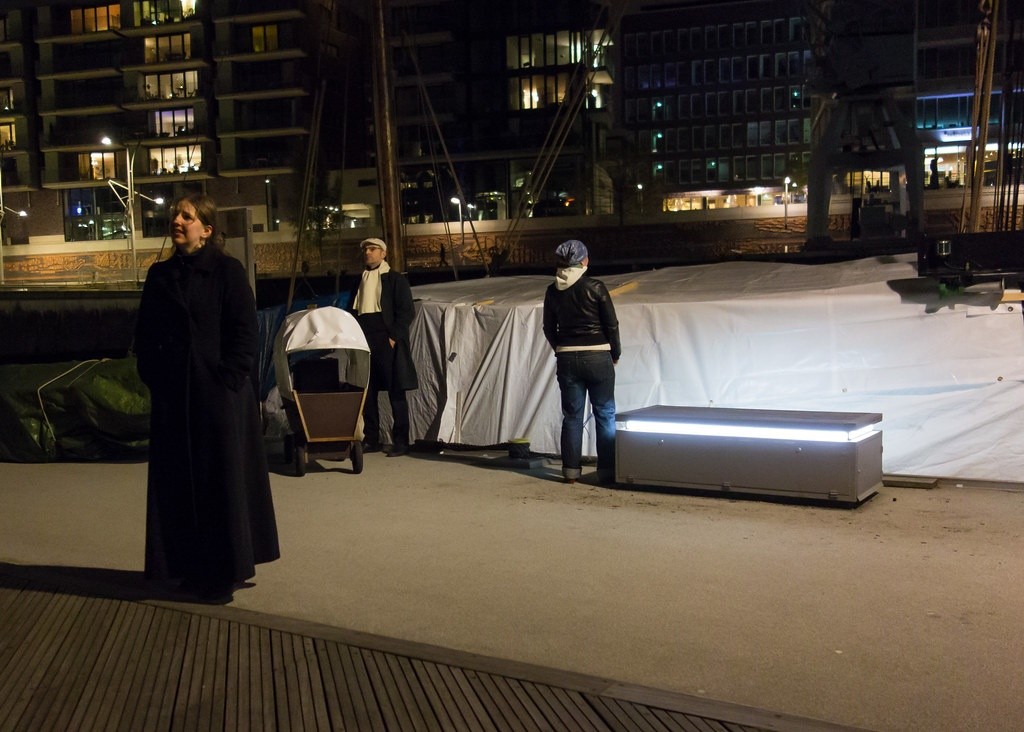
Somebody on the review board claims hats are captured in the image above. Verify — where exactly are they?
[555,240,587,267]
[360,237,386,252]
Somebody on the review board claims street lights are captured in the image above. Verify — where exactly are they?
[785,177,791,228]
[102,136,163,291]
[452,198,464,250]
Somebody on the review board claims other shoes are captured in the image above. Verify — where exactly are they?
[388,443,408,457]
[566,477,577,483]
[359,441,379,452]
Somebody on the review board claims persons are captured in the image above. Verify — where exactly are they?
[343,237,415,457]
[135,192,281,602]
[541,239,622,484]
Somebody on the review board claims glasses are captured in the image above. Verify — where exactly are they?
[362,247,381,252]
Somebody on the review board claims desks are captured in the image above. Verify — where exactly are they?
[614,404,884,504]
